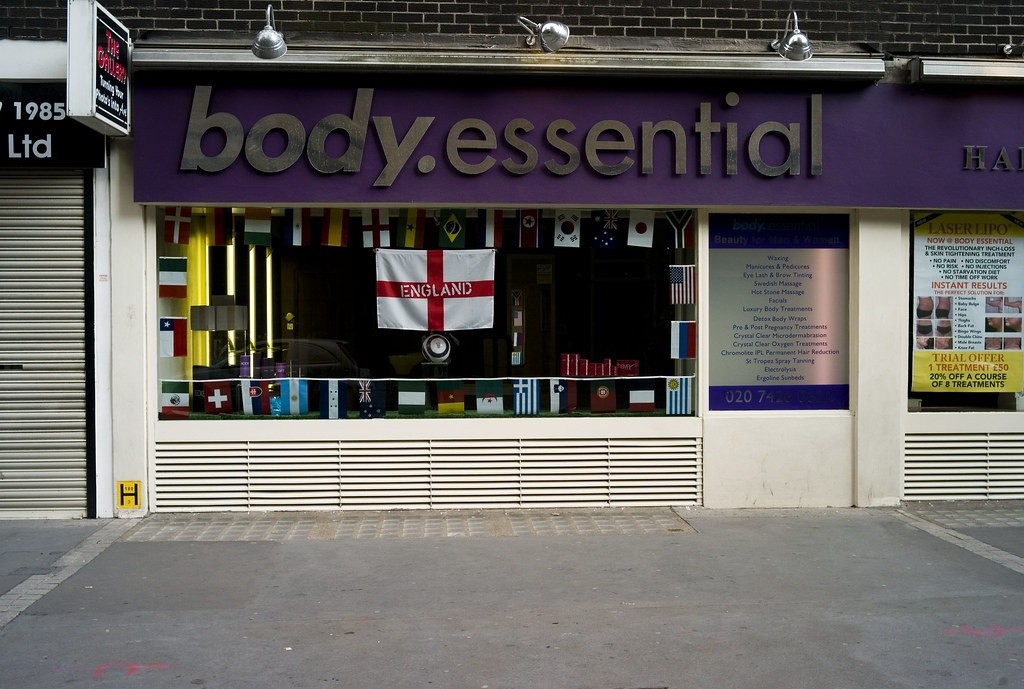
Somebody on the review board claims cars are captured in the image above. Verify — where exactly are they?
[194,337,397,382]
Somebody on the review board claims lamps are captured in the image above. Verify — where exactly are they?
[517,16,570,53]
[251,4,287,60]
[770,10,814,62]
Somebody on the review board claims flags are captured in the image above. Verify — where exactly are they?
[355,381,387,419]
[319,381,349,419]
[512,379,542,416]
[473,380,504,415]
[590,380,616,413]
[549,379,577,414]
[375,247,495,331]
[279,379,308,417]
[510,290,524,365]
[666,377,692,415]
[669,320,697,359]
[397,381,426,415]
[161,381,190,419]
[629,378,655,413]
[157,256,187,300]
[202,382,234,414]
[668,265,694,305]
[240,380,271,415]
[435,381,465,416]
[158,316,186,359]
[164,205,696,248]
